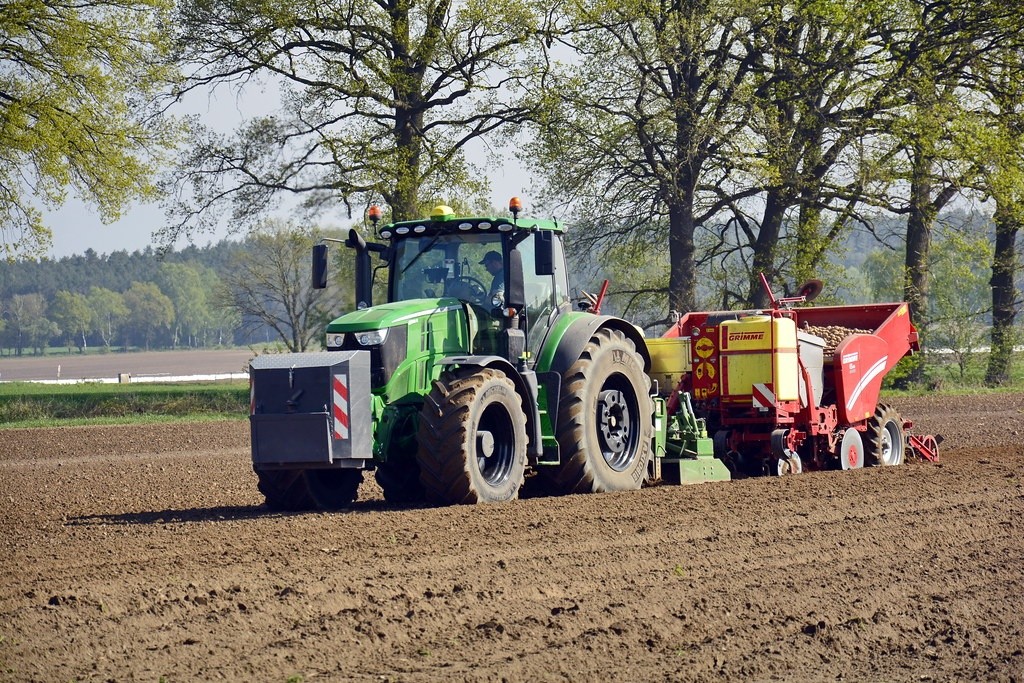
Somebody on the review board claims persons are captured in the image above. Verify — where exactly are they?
[478,250,505,307]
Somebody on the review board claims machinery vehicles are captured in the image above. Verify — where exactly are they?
[248,197,730,506]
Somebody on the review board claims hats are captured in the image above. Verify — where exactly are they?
[478,251,502,265]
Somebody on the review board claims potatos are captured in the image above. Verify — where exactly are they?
[798,326,873,357]
